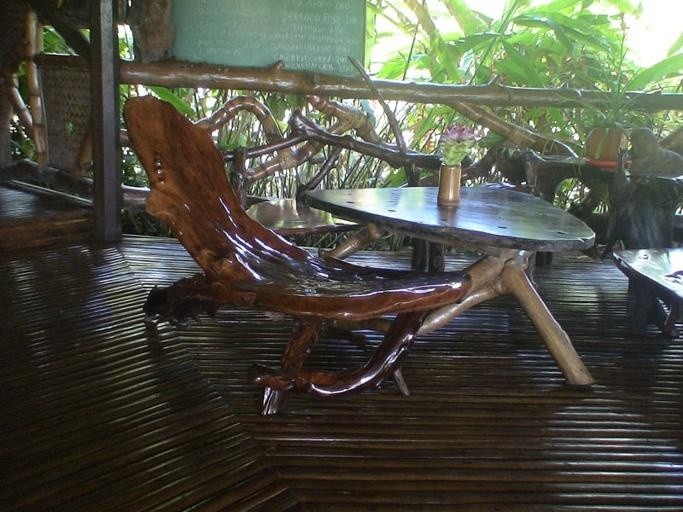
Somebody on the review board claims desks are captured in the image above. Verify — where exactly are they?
[299,183,601,391]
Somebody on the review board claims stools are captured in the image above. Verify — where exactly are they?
[611,244,683,340]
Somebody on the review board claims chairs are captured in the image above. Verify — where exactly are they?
[110,79,472,414]
